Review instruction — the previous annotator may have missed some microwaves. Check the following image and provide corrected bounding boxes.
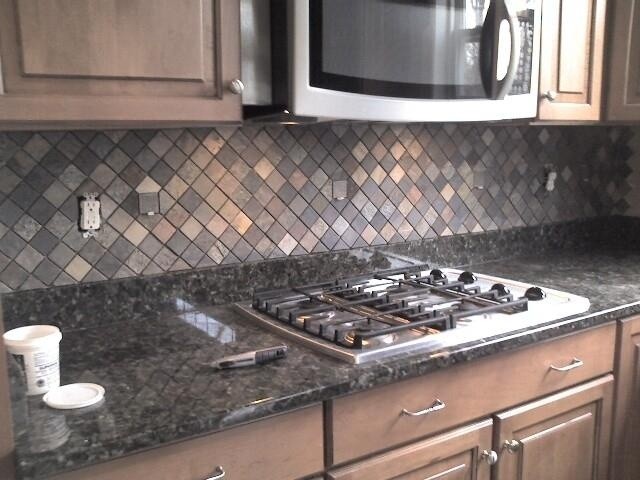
[251,0,543,125]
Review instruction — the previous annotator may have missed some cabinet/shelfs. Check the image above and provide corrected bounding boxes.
[329,322,616,480]
[1,0,246,130]
[70,400,325,476]
[528,0,638,127]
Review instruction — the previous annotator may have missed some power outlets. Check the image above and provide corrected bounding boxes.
[77,195,104,234]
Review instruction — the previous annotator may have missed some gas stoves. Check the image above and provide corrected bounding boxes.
[230,264,591,366]
